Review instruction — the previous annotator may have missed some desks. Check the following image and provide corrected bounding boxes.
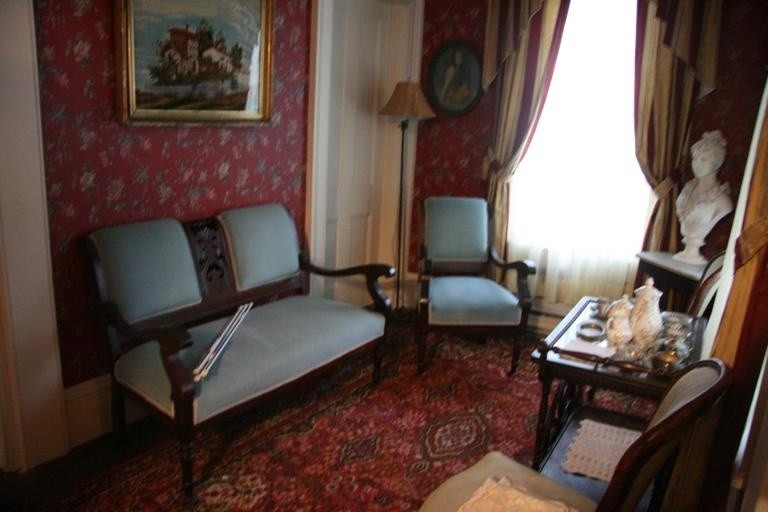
[637,251,707,297]
[528,294,707,501]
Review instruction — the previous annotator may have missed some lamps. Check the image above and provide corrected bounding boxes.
[373,80,436,323]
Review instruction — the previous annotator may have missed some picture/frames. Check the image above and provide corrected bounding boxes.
[425,40,484,117]
[110,1,275,127]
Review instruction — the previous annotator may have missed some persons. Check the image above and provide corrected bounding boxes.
[675,130,733,246]
[439,49,471,110]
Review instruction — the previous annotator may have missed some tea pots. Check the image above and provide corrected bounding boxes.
[630,277,663,346]
[604,292,634,348]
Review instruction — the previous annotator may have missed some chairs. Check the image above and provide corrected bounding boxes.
[413,194,536,378]
[413,357,729,511]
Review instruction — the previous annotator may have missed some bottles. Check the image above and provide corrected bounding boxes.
[654,312,694,374]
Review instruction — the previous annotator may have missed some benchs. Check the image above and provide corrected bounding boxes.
[77,199,397,502]
